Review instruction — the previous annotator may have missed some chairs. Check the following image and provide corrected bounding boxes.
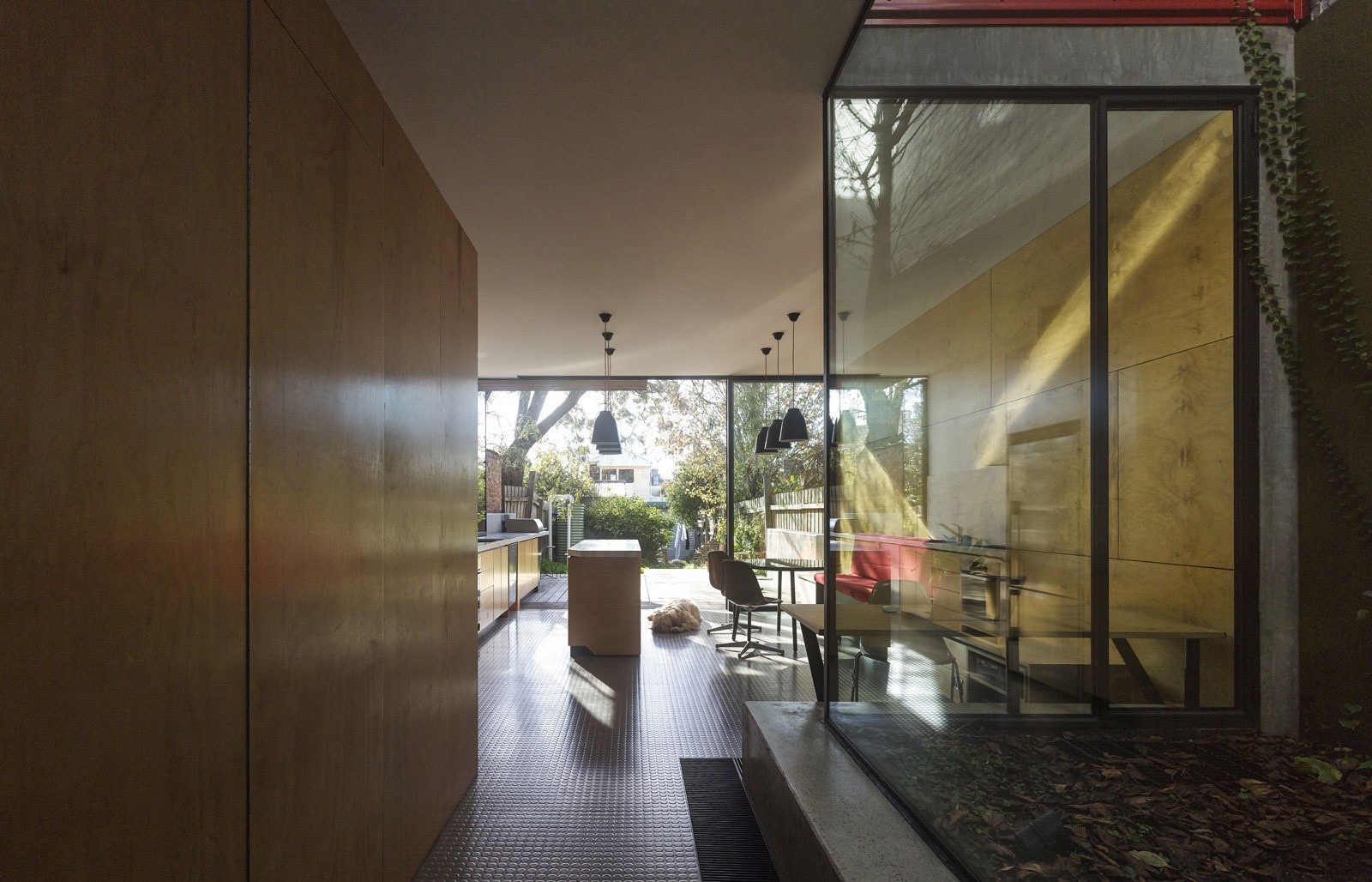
[707,551,762,641]
[850,580,963,705]
[715,559,785,658]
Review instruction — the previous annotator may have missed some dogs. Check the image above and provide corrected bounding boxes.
[648,598,703,633]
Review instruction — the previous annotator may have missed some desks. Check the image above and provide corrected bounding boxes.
[736,557,842,653]
[779,604,1228,712]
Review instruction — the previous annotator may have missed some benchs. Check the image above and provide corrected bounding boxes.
[814,532,943,605]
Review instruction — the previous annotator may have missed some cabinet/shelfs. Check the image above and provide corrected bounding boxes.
[477,544,510,634]
[512,538,538,610]
[899,544,1007,656]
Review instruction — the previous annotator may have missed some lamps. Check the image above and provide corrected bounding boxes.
[590,313,623,456]
[778,312,808,442]
[764,332,791,451]
[754,347,779,455]
